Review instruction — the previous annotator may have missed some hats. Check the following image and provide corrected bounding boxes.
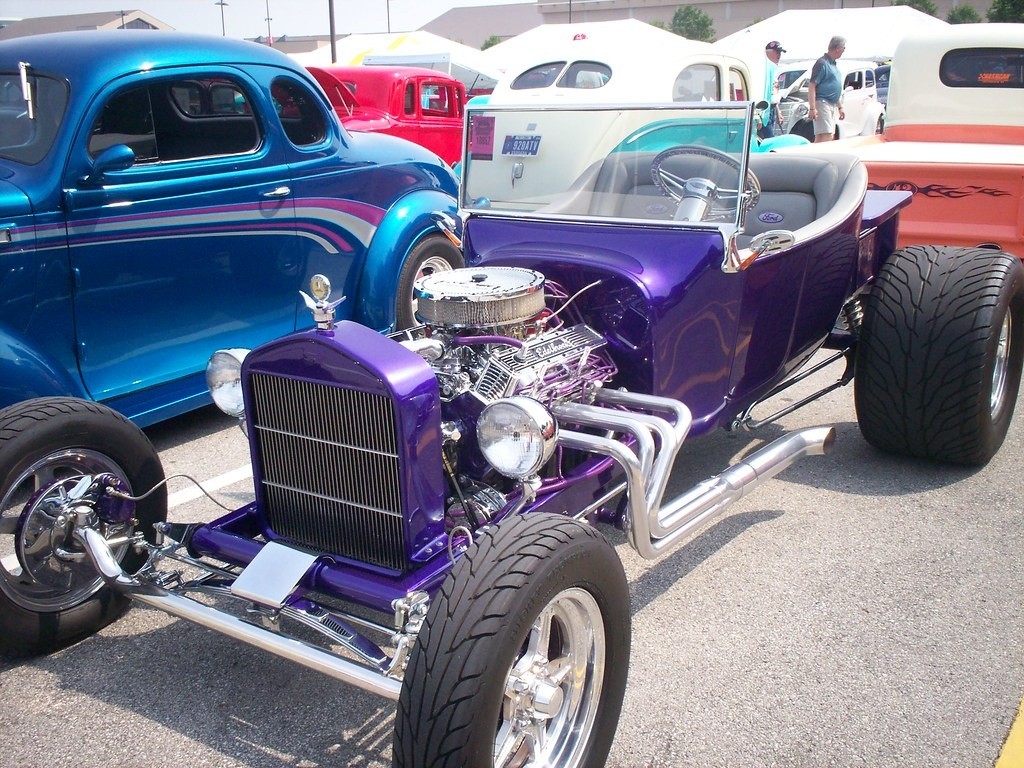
[766,41,787,53]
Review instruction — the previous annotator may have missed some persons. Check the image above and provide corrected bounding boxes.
[751,41,786,140]
[808,36,846,144]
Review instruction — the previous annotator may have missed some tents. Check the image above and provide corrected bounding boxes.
[283,4,953,75]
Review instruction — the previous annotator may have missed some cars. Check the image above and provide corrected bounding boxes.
[1,92,1022,768]
[286,15,1024,291]
[1,27,492,481]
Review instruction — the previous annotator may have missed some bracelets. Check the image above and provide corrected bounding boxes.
[839,107,844,110]
[810,109,815,110]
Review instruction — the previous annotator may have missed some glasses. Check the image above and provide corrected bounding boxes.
[841,47,845,49]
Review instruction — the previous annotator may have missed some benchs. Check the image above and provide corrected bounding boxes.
[592,149,868,256]
